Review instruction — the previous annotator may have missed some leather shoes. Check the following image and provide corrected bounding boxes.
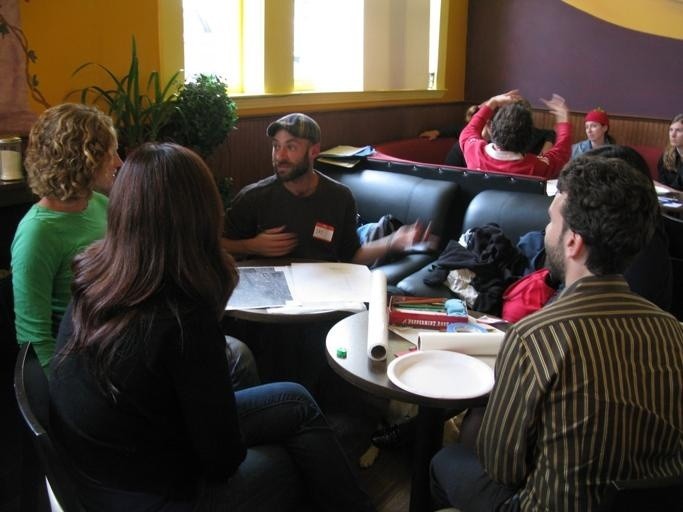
[371,417,418,449]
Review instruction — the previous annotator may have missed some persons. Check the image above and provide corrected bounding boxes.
[568,107,616,162]
[656,112,683,191]
[442,104,490,168]
[457,89,573,181]
[47,140,381,512]
[218,109,434,415]
[10,101,262,393]
[429,156,683,510]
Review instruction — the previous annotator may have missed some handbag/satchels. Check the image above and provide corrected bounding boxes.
[502,268,556,323]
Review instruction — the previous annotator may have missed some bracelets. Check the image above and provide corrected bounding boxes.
[385,231,394,255]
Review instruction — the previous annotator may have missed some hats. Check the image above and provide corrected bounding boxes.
[267,113,321,143]
[584,108,609,125]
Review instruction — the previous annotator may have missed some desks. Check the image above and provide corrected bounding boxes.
[205,248,371,321]
[325,302,519,512]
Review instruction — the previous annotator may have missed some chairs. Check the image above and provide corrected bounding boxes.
[12,339,98,512]
[312,171,554,299]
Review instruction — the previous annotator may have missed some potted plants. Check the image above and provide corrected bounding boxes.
[62,33,184,162]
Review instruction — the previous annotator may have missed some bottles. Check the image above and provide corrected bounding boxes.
[1,134,25,182]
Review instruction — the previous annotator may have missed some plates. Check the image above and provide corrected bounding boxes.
[387,349,496,401]
